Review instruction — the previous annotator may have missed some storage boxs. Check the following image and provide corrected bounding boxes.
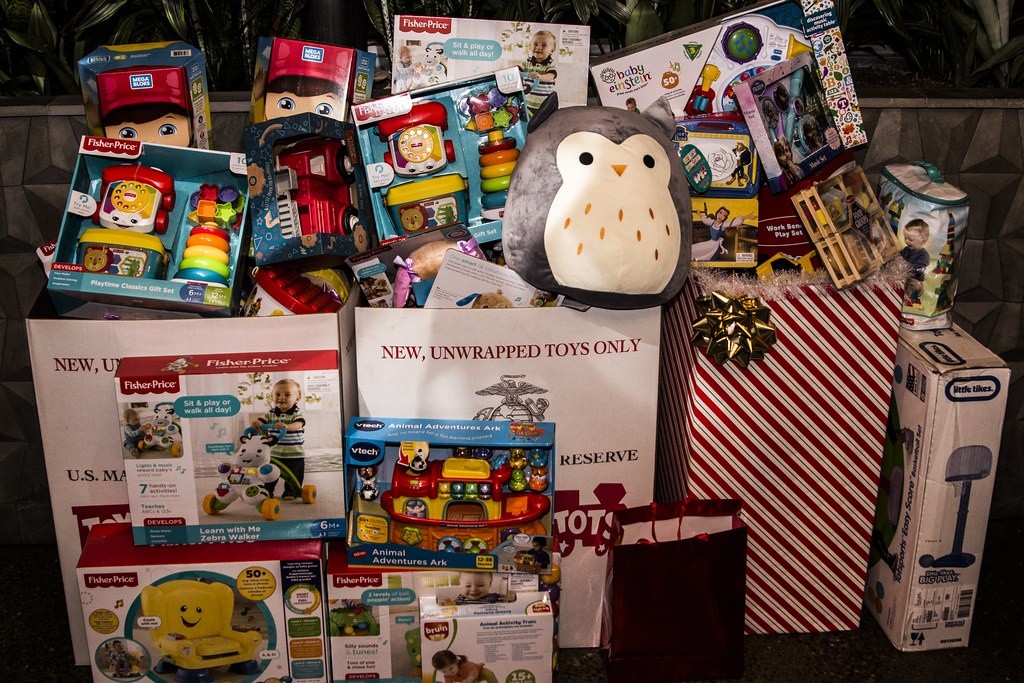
[24,0,1013,683]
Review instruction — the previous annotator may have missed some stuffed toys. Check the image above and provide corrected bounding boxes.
[500,91,693,310]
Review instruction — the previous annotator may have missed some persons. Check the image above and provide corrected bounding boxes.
[111,640,136,676]
[518,536,551,569]
[453,571,508,606]
[394,47,422,94]
[432,650,487,683]
[898,218,931,300]
[516,31,558,120]
[123,409,160,459]
[252,379,306,501]
[626,98,641,115]
